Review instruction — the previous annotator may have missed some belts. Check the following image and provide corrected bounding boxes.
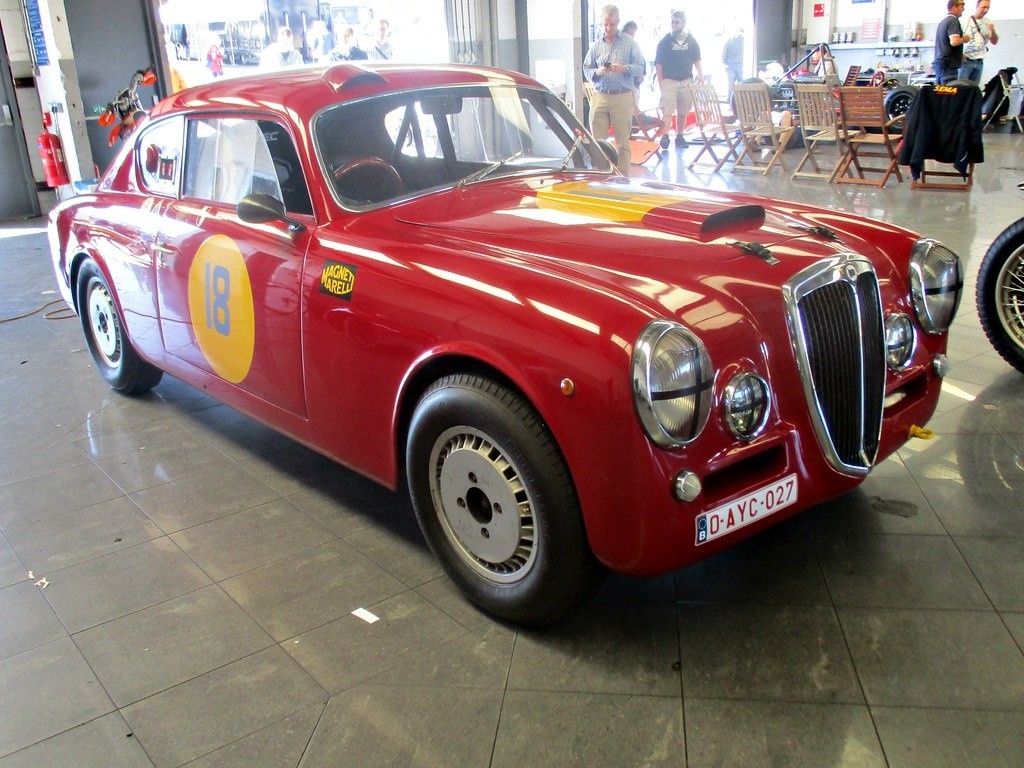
[596,88,630,94]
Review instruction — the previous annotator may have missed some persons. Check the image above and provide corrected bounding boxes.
[721,25,743,103]
[621,20,647,134]
[958,0,999,85]
[931,0,971,85]
[205,45,225,79]
[582,4,645,177]
[653,11,704,149]
[810,43,838,77]
[260,19,397,70]
[746,110,821,155]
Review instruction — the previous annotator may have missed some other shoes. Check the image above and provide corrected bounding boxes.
[771,142,785,154]
[751,141,762,153]
[675,134,689,148]
[661,135,670,149]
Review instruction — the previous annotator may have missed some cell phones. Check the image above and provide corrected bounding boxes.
[604,62,611,68]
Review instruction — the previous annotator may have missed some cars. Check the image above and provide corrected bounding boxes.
[47,62,964,626]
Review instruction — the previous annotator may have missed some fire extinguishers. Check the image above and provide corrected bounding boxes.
[36,124,69,186]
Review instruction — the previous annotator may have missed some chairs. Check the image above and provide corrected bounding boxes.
[687,82,751,173]
[318,110,428,200]
[981,67,1024,135]
[790,83,861,184]
[582,81,665,162]
[842,65,861,86]
[835,86,908,188]
[730,83,795,176]
[909,85,979,192]
[226,133,311,215]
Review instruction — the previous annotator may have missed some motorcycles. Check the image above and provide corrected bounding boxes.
[98,64,159,147]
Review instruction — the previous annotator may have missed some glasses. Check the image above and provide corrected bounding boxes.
[672,20,679,25]
[956,2,965,6]
